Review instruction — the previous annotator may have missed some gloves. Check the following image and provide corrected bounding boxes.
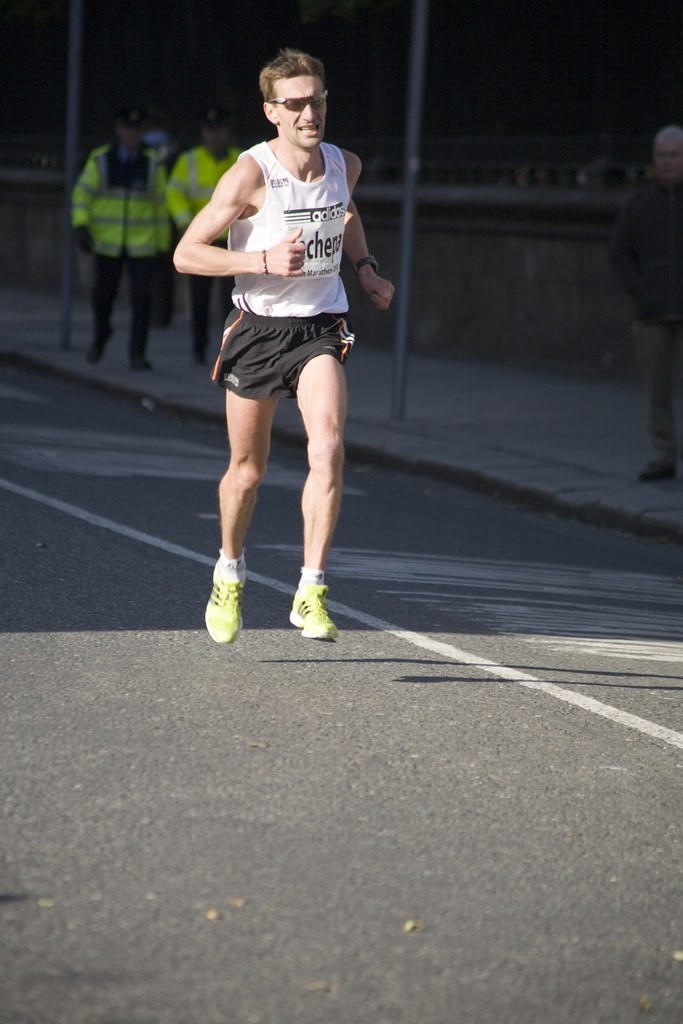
[76,226,95,254]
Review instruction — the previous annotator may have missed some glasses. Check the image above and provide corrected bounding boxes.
[267,89,328,111]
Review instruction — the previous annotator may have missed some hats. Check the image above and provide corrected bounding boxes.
[193,104,232,127]
[112,105,147,127]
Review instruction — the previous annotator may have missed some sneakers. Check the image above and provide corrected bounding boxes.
[205,559,247,644]
[289,583,338,639]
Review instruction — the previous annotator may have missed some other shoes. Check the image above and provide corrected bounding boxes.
[129,356,150,370]
[192,333,209,364]
[640,464,677,480]
[86,328,113,363]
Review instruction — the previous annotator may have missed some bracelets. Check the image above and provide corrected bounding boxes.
[354,255,378,272]
[262,250,268,275]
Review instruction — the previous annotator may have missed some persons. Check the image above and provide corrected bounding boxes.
[173,52,395,643]
[613,127,683,482]
[69,110,169,370]
[164,112,245,367]
[141,130,180,330]
